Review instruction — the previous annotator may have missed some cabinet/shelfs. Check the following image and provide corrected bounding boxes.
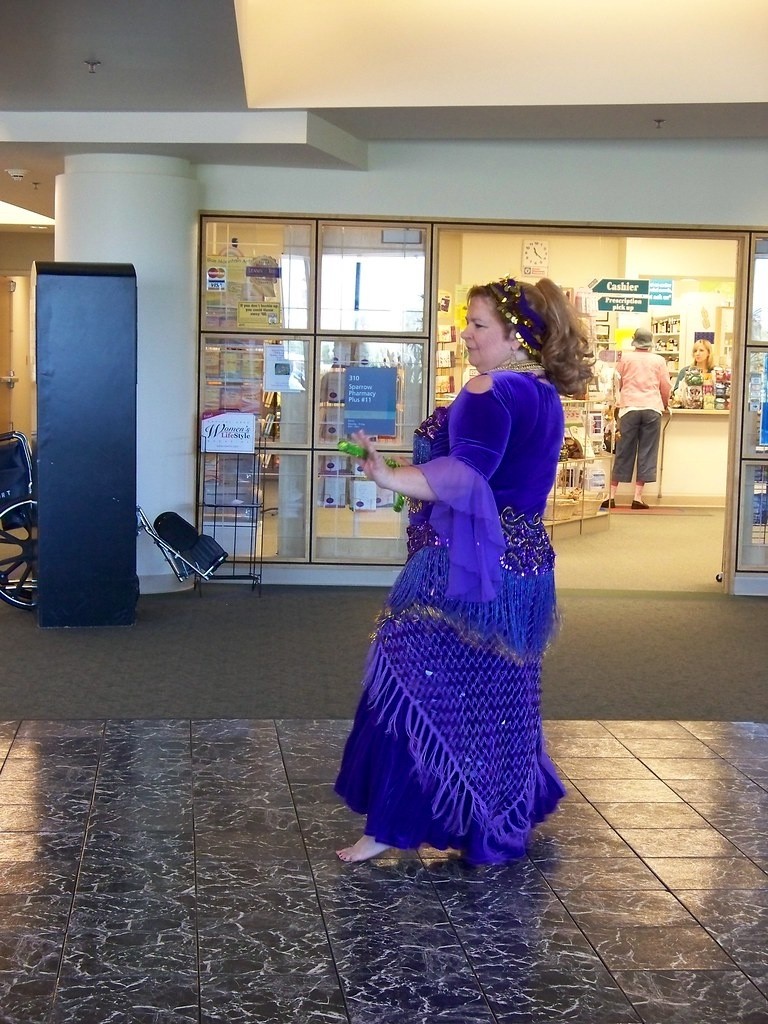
[542,342,617,541]
[193,434,267,600]
[203,296,263,556]
[650,311,696,375]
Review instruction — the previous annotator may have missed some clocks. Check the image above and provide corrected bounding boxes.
[521,240,549,278]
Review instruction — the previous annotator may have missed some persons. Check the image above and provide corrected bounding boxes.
[333,276,599,865]
[672,339,720,394]
[601,328,671,510]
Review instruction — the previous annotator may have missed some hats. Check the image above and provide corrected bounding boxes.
[629,327,654,348]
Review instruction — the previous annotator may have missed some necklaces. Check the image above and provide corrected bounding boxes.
[493,360,545,371]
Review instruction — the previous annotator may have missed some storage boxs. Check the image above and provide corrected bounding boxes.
[436,324,456,344]
[205,280,263,523]
[321,372,345,403]
[318,455,347,476]
[200,409,260,456]
[436,350,456,368]
[435,376,455,394]
[347,457,366,481]
[349,480,377,513]
[319,406,347,444]
[377,486,394,508]
[317,477,346,509]
[348,433,380,444]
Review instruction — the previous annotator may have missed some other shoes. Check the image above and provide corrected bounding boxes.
[632,499,650,509]
[601,499,616,509]
